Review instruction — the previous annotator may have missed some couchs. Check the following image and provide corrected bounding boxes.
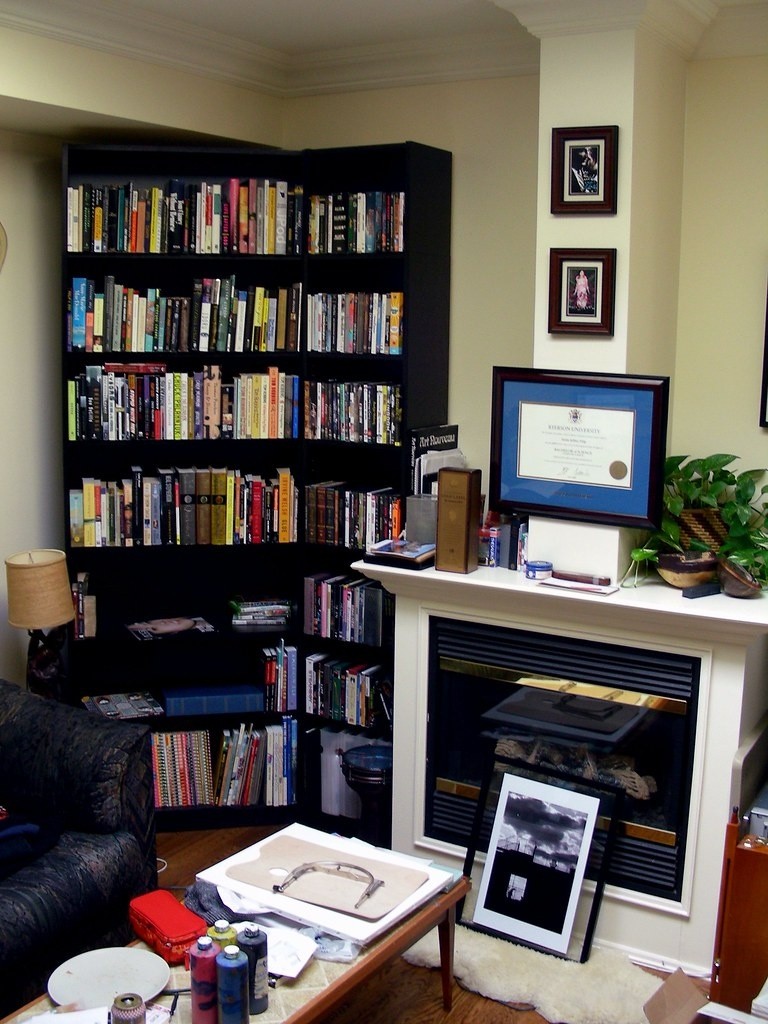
[0,682,156,1023]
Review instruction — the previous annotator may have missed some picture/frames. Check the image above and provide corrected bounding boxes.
[550,126,618,217]
[453,752,625,966]
[548,246,617,337]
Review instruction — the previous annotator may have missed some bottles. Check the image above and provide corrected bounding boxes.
[189,920,269,1024]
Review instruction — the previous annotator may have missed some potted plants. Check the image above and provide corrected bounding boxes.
[626,453,768,589]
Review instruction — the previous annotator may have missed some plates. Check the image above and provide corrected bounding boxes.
[47,946,171,1009]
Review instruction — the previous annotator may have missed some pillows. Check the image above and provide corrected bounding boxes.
[0,798,55,881]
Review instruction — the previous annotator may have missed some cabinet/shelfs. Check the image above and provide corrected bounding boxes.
[64,130,457,833]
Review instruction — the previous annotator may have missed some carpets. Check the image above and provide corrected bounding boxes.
[404,924,663,1024]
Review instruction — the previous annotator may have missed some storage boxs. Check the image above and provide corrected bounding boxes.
[434,467,482,575]
[406,491,437,543]
[129,891,208,970]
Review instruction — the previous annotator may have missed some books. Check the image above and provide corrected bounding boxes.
[230,598,291,625]
[68,363,403,446]
[126,616,219,641]
[67,177,405,255]
[304,571,396,646]
[151,714,297,807]
[70,466,400,556]
[81,691,164,720]
[71,572,96,639]
[362,424,464,570]
[263,638,393,742]
[489,513,528,573]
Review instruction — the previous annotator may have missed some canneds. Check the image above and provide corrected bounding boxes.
[110,993,147,1024]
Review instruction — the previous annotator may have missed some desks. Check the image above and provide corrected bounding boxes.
[0,866,472,1024]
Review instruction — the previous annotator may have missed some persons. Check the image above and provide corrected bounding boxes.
[573,270,590,309]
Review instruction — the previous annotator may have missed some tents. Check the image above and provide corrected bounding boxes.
[67,275,403,354]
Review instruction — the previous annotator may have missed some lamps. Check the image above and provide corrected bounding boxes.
[4,549,73,698]
